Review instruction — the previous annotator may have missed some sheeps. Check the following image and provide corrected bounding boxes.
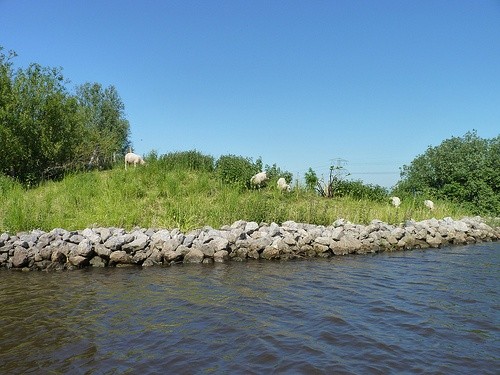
[390,196,403,208]
[424,198,435,211]
[124,152,146,171]
[251,171,270,190]
[276,177,293,195]
